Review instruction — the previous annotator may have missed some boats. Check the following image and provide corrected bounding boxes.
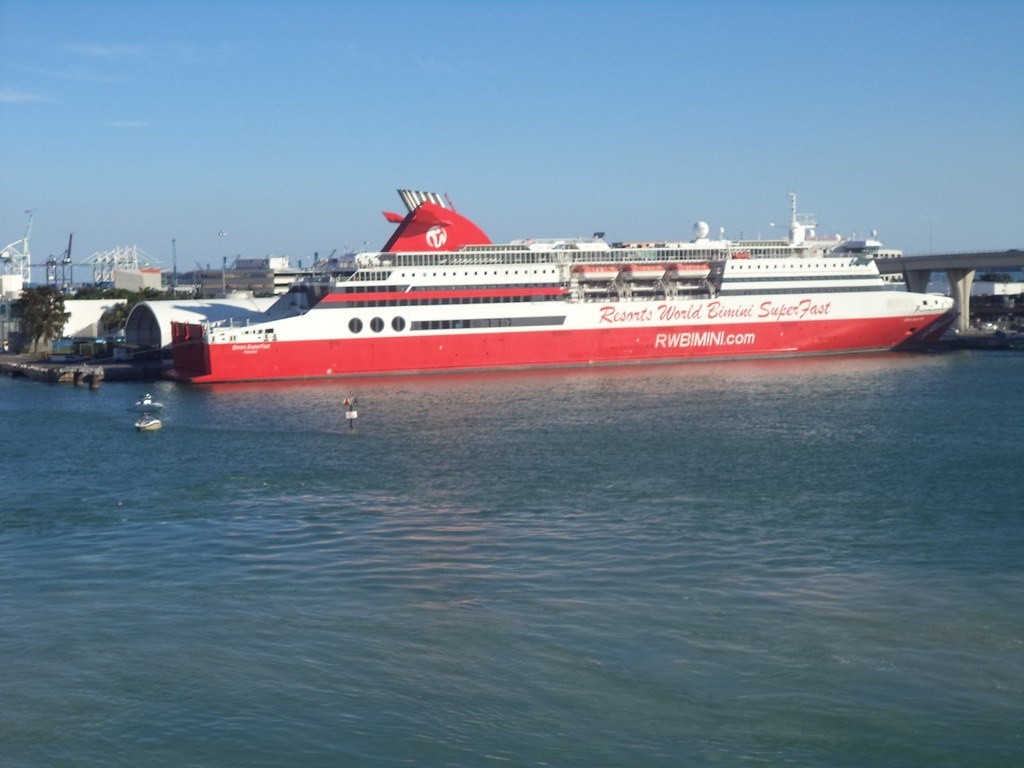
[151,189,955,387]
[135,412,163,431]
[129,392,163,413]
